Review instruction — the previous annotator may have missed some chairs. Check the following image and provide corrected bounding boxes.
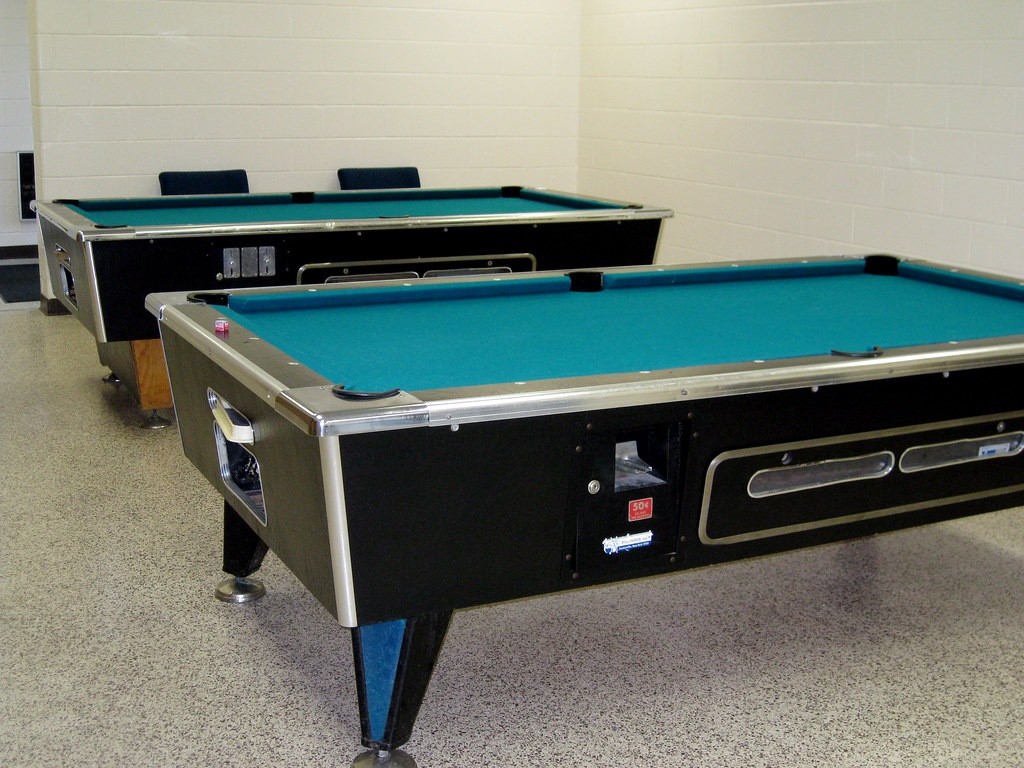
[338,164,423,191]
[158,167,248,197]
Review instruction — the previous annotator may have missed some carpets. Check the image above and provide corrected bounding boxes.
[0,263,40,304]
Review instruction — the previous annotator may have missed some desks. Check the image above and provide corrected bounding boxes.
[23,182,676,430]
[140,247,1024,768]
